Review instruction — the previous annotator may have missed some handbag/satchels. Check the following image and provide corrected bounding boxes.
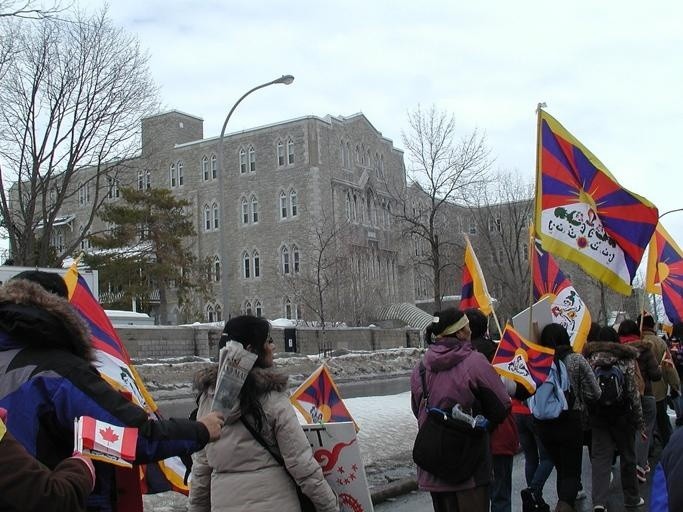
[412,409,486,483]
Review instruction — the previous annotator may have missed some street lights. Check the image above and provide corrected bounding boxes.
[217,75,295,325]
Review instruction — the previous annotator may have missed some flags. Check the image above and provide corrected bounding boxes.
[60,264,191,494]
[290,363,359,433]
[73,412,141,470]
[529,223,593,355]
[535,107,659,297]
[458,244,495,319]
[644,219,683,326]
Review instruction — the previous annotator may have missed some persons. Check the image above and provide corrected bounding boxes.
[0,407,98,512]
[183,315,340,511]
[1,268,227,512]
[408,304,683,511]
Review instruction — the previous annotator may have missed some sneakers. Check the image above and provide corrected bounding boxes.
[521,488,608,512]
[624,497,645,506]
[636,463,651,482]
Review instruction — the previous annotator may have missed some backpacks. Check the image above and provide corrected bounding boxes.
[527,358,575,421]
[589,359,628,415]
